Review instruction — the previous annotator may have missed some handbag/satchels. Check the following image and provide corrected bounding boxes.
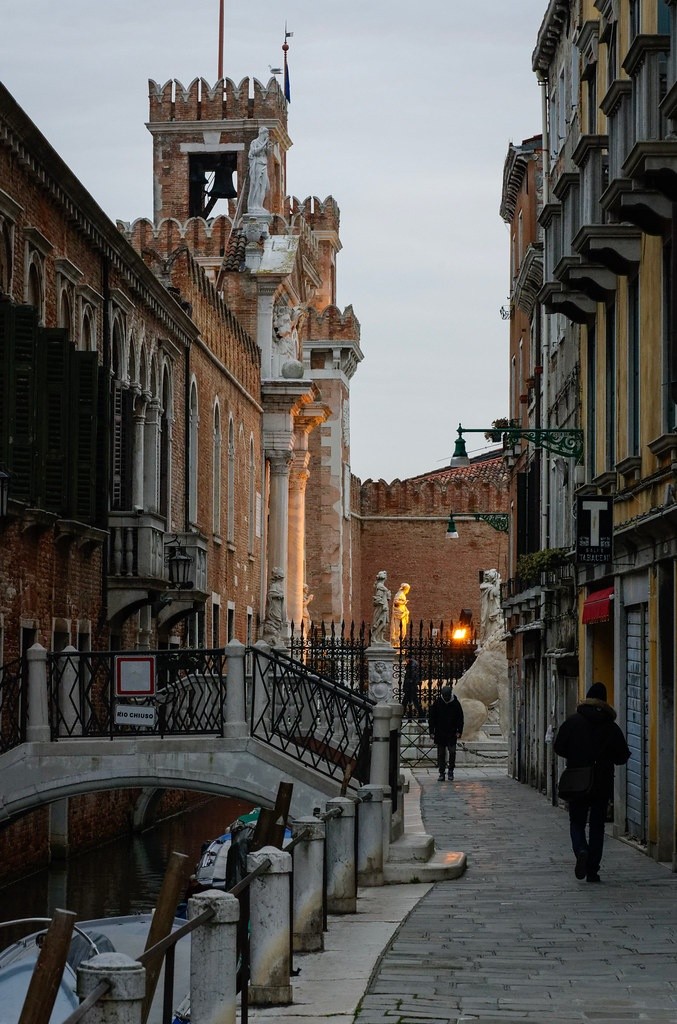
[558,766,600,802]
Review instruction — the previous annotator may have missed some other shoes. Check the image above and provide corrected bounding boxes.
[448,771,454,780]
[587,875,600,882]
[437,772,446,780]
[575,850,588,879]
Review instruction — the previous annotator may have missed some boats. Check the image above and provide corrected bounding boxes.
[0,912,194,1023]
[193,805,295,893]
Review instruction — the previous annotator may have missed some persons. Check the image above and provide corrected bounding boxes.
[477,568,504,646]
[273,305,300,377]
[372,571,410,647]
[401,654,425,721]
[247,126,272,215]
[428,686,465,781]
[553,682,632,881]
[301,583,313,640]
[262,567,286,650]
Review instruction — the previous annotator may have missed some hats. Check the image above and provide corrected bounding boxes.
[586,682,607,703]
